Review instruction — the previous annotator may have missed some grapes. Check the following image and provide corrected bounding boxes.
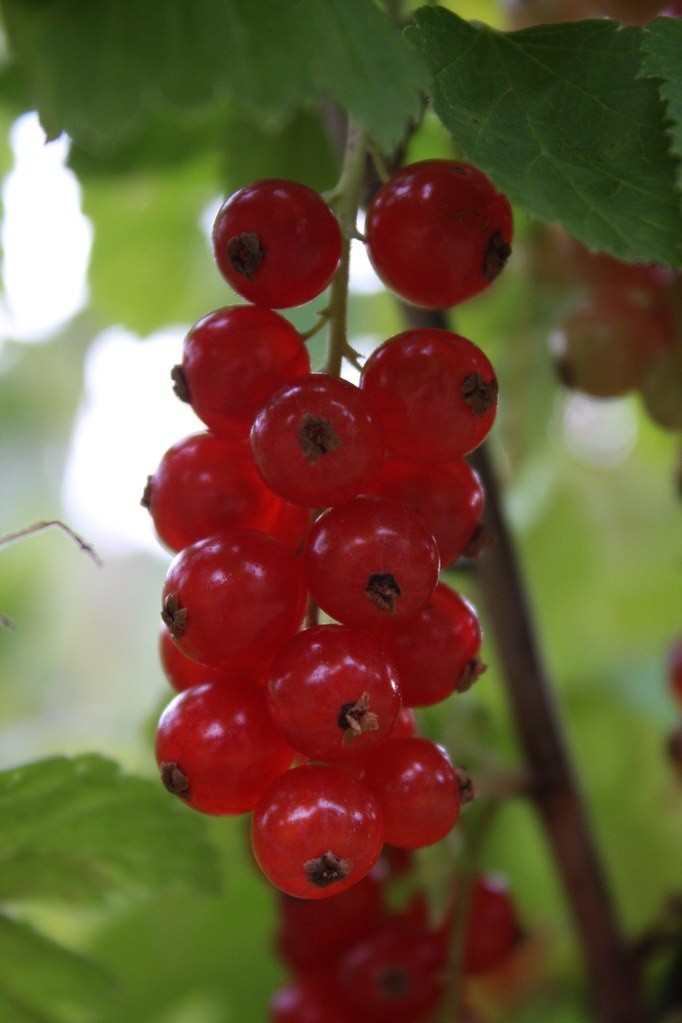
[141,156,682,1022]
